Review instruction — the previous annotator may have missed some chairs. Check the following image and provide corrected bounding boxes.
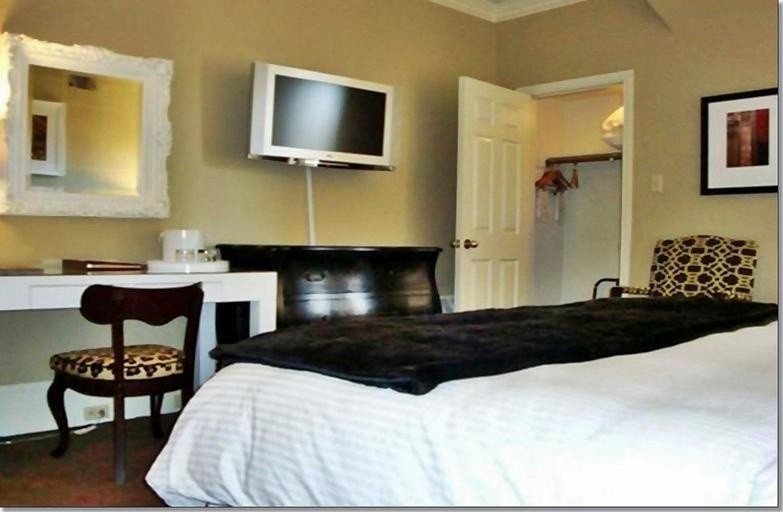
[43,284,203,487]
[608,233,758,302]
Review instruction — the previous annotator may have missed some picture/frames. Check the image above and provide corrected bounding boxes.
[701,86,777,194]
[31,100,65,176]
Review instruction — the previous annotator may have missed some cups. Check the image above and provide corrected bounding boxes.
[197,249,222,265]
[175,249,195,265]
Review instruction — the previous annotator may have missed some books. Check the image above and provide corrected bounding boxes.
[62,259,147,272]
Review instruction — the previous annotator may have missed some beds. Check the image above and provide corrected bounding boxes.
[143,297,778,506]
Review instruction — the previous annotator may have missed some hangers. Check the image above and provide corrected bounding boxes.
[535,166,580,198]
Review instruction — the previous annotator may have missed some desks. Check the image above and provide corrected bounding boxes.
[0,268,279,392]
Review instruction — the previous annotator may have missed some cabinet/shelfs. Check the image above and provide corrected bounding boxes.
[209,243,442,372]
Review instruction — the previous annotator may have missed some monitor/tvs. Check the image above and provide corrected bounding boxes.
[249,61,401,168]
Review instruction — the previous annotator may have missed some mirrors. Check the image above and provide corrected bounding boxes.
[1,29,175,219]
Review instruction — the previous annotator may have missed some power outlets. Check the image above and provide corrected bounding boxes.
[83,403,109,422]
